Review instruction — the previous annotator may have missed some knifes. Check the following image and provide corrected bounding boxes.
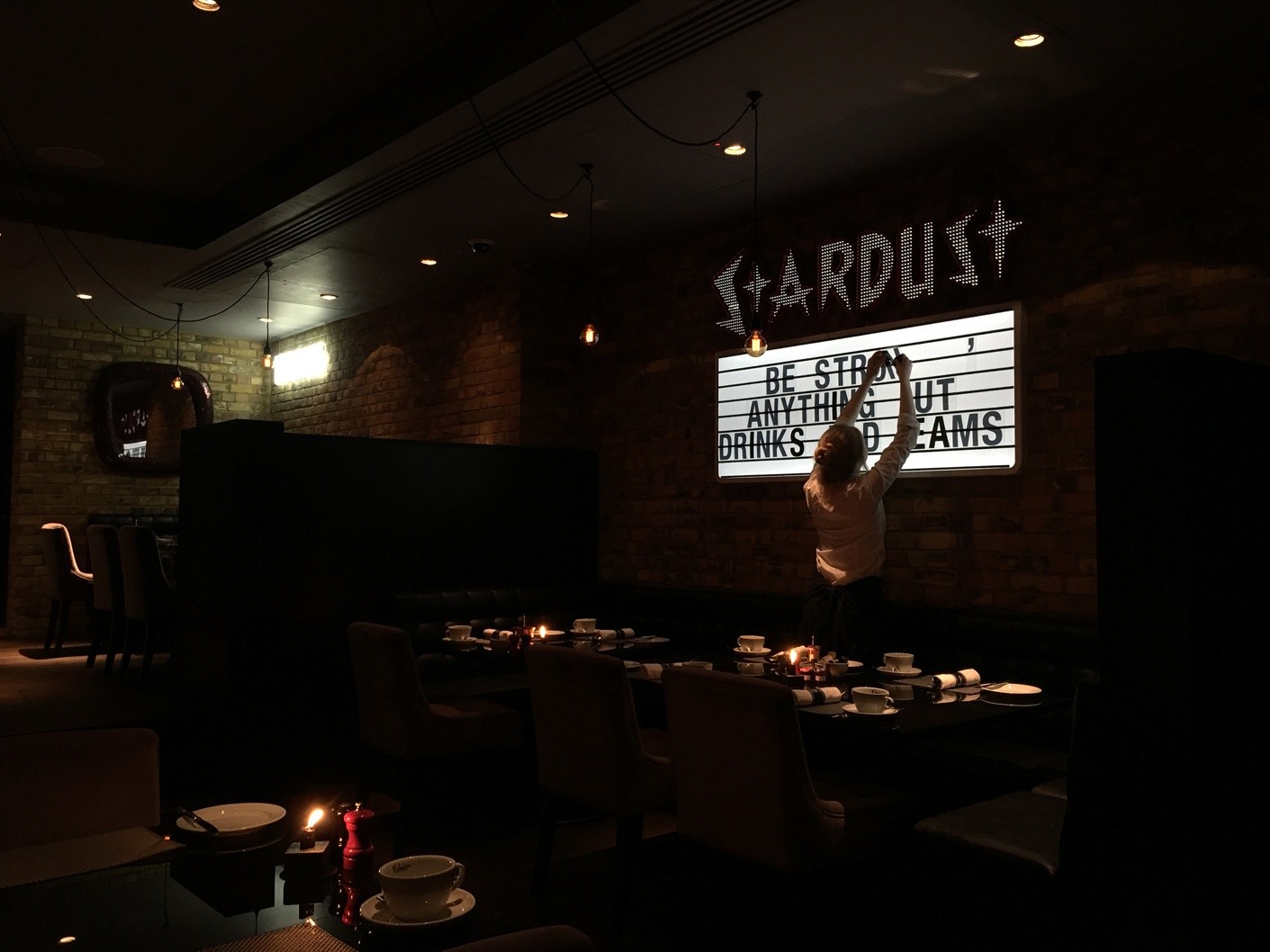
[175,806,219,833]
[975,680,1009,688]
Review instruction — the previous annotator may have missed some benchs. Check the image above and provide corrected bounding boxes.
[88,511,178,647]
[365,583,1100,952]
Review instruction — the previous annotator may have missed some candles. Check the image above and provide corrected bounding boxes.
[300,803,326,849]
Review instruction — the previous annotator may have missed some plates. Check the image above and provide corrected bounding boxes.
[842,703,899,715]
[733,656,778,664]
[980,683,1042,695]
[732,647,772,656]
[632,636,671,643]
[876,666,922,677]
[570,629,600,636]
[978,693,1043,707]
[817,660,864,668]
[878,675,915,687]
[442,637,477,641]
[176,802,286,837]
[359,888,476,926]
[621,660,641,668]
[533,630,565,638]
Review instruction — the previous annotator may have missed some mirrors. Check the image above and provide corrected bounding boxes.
[96,361,214,476]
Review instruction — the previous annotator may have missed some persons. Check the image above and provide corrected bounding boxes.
[802,351,918,657]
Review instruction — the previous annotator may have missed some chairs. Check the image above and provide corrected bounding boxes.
[0,727,161,851]
[41,523,178,689]
[348,620,925,952]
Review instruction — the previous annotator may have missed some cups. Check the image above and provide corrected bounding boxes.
[737,662,764,676]
[737,635,765,652]
[378,854,466,922]
[884,684,914,700]
[883,652,914,672]
[445,625,472,640]
[573,619,596,632]
[851,686,895,712]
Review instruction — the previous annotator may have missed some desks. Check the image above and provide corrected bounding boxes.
[412,631,1080,795]
[0,797,493,952]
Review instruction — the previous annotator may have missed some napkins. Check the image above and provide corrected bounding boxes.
[640,660,682,679]
[792,685,839,706]
[597,627,634,641]
[934,668,982,693]
[481,626,513,639]
